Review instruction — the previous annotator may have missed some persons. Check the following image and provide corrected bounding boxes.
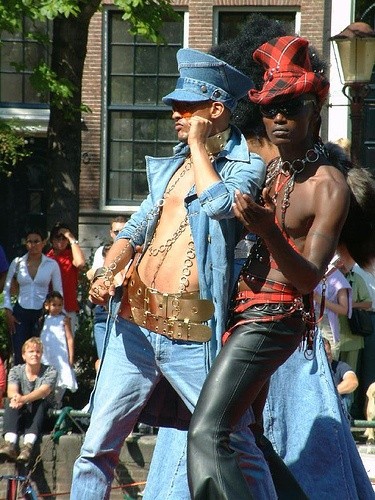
[68,47,278,500]
[333,256,372,372]
[311,261,348,360]
[336,241,375,420]
[0,337,58,463]
[40,291,78,409]
[87,215,133,375]
[45,227,85,339]
[322,337,358,425]
[2,229,63,365]
[187,36,353,500]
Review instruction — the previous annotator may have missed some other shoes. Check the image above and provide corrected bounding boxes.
[17,443,33,464]
[0,440,18,460]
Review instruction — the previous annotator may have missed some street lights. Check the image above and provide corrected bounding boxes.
[327,22,375,167]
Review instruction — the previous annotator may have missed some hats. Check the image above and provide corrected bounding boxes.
[162,48,256,114]
[248,35,330,104]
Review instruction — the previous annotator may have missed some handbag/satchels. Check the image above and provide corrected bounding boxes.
[10,257,20,297]
[347,308,375,337]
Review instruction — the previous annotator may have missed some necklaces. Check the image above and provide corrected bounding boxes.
[93,124,235,337]
[234,136,335,292]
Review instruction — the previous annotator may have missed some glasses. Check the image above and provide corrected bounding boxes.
[111,230,120,236]
[26,239,42,246]
[52,234,67,240]
[170,98,212,115]
[259,97,316,118]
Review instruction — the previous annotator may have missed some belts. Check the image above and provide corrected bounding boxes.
[119,266,215,343]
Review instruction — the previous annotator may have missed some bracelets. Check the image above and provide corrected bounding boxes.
[69,239,79,244]
[92,267,114,285]
